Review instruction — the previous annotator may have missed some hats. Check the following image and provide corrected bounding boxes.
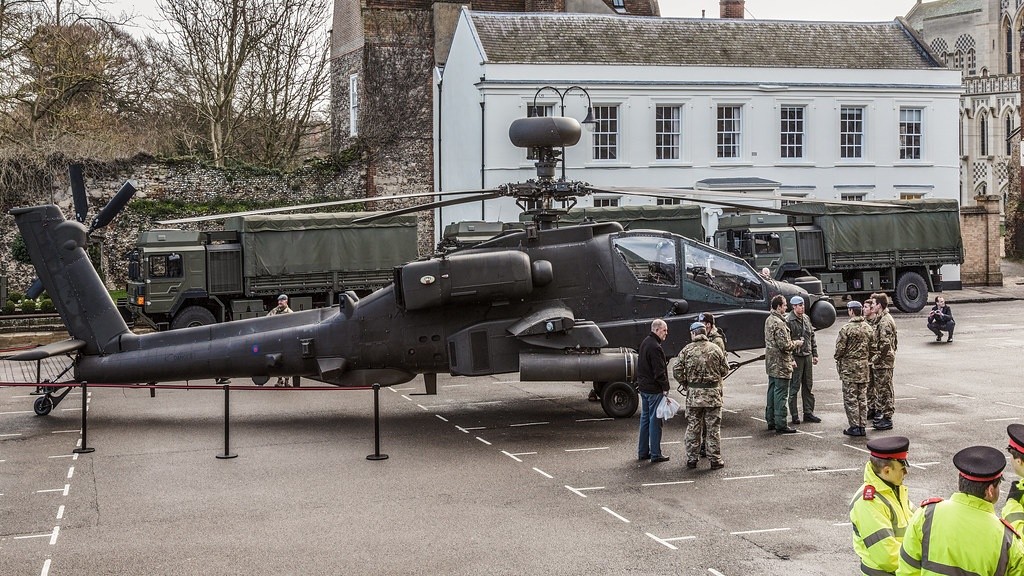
[690,322,706,331]
[867,436,910,468]
[953,446,1007,482]
[790,296,804,304]
[847,301,863,308]
[277,295,288,300]
[1005,423,1024,454]
[699,313,715,324]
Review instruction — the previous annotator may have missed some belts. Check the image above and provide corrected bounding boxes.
[688,381,719,388]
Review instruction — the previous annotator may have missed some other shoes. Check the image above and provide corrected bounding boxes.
[947,337,953,342]
[792,416,800,424]
[803,413,821,423]
[711,461,724,470]
[936,333,944,341]
[873,417,893,429]
[687,460,696,468]
[768,425,776,430]
[876,410,882,416]
[867,409,877,419]
[639,454,651,459]
[275,383,292,387]
[651,456,670,462]
[843,427,866,436]
[776,427,796,433]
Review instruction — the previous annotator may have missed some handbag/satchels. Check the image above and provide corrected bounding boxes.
[656,396,680,418]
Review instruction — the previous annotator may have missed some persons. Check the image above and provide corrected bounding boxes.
[895,446,1024,576]
[849,436,918,576]
[266,294,294,387]
[1001,424,1024,545]
[765,295,821,433]
[673,312,730,470]
[638,319,670,462]
[927,296,955,342]
[834,293,897,436]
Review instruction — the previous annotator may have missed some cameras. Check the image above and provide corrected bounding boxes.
[931,310,940,314]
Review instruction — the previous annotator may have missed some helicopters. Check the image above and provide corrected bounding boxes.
[8,116,831,416]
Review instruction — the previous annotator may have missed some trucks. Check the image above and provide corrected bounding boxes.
[713,197,965,314]
[126,210,420,331]
[436,204,706,253]
